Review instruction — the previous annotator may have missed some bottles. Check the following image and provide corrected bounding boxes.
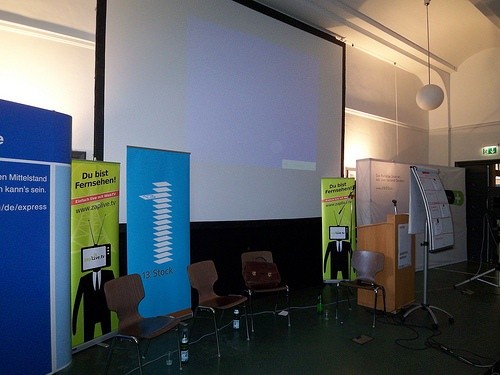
[316,293,323,315]
[181,332,190,363]
[232,306,240,330]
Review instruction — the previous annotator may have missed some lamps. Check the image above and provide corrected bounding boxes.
[415,0,445,112]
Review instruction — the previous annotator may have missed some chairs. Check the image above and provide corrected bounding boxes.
[333,249,389,330]
[240,251,291,332]
[186,260,251,357]
[105,273,181,375]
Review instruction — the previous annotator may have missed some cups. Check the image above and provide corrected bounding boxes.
[323,308,329,320]
[166,350,173,365]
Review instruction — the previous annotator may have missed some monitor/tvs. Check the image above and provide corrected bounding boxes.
[329,210,348,240]
[80,215,111,272]
